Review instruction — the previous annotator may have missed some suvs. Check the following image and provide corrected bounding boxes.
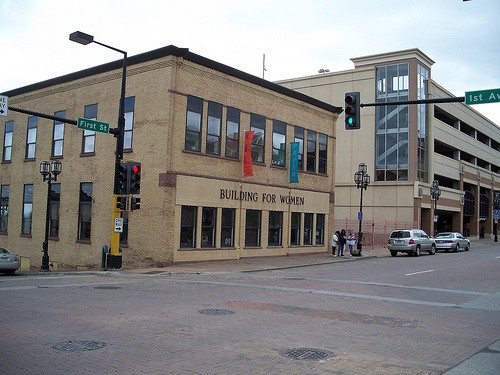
[386,228,436,257]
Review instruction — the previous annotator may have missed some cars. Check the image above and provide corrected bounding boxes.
[434,232,471,253]
[0,247,20,274]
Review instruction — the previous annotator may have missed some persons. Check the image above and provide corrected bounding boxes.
[348,232,356,256]
[338,229,347,256]
[331,231,339,256]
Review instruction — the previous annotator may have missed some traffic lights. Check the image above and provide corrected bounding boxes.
[126,161,142,195]
[344,92,360,130]
[116,196,127,212]
[113,162,127,195]
[128,197,141,212]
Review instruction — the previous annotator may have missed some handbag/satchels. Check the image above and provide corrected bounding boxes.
[347,239,357,246]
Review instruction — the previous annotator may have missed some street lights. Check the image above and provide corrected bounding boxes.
[493,194,500,242]
[39,160,63,272]
[354,162,371,256]
[430,180,442,238]
[69,30,128,270]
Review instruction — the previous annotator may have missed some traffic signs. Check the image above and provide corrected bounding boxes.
[114,218,123,233]
[0,95,8,117]
